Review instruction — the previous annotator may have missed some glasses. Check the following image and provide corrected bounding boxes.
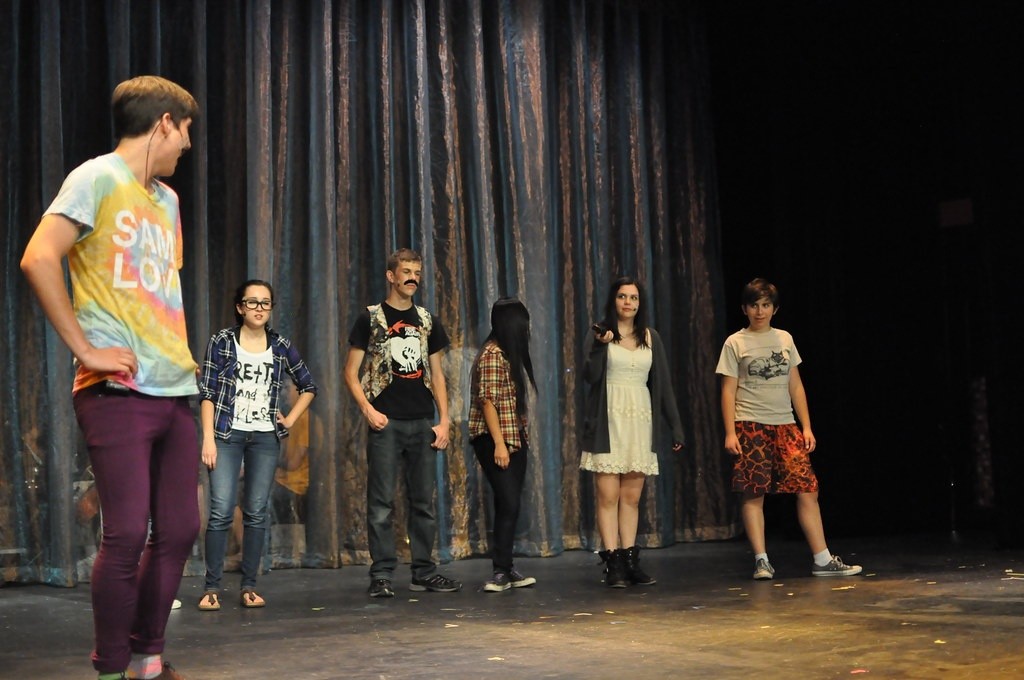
[241,298,273,311]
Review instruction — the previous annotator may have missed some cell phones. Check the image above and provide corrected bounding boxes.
[591,324,606,336]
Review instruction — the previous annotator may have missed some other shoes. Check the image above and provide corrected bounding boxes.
[128,661,187,680]
[171,599,181,609]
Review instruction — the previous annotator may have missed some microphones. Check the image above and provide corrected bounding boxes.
[633,308,637,311]
[397,280,400,285]
[243,312,246,316]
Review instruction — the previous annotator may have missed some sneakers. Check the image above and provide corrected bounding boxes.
[369,579,396,597]
[812,554,867,577]
[482,572,512,591]
[754,559,774,579]
[509,570,537,587]
[408,570,462,591]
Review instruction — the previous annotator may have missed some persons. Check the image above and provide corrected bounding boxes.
[714,279,865,580]
[345,249,461,597]
[576,278,687,587]
[199,280,317,609]
[20,74,200,680]
[469,296,537,592]
[232,379,323,558]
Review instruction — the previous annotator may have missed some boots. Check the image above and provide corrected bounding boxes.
[628,545,657,586]
[599,548,630,589]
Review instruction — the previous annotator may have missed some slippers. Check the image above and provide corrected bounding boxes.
[240,585,266,608]
[199,590,221,611]
